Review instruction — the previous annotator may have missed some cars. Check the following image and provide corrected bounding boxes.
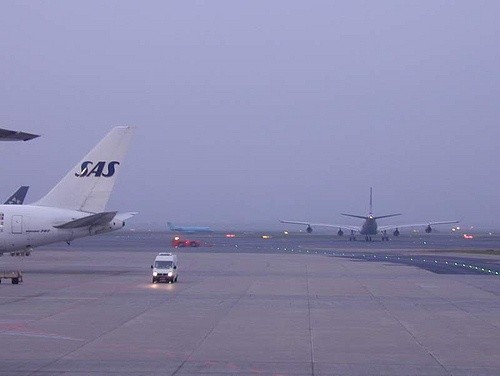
[174,235,200,248]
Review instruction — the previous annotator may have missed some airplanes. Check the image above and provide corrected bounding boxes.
[1,124,139,284]
[166,222,215,235]
[279,187,459,244]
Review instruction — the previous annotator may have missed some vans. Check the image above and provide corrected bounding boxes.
[150,252,178,283]
